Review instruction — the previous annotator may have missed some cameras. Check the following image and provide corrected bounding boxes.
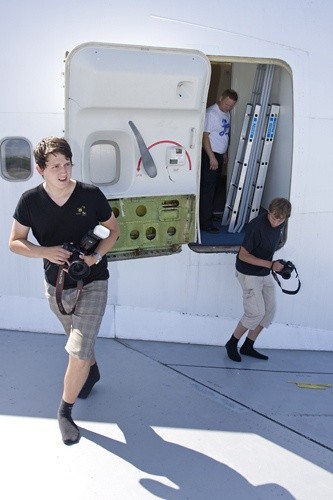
[62,224,110,282]
[276,259,295,279]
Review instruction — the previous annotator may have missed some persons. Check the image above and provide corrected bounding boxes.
[8,135,121,445]
[199,89,238,234]
[225,198,294,362]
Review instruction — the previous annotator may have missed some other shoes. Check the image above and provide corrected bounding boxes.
[208,214,222,222]
[200,225,220,233]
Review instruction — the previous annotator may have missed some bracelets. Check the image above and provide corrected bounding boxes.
[270,261,276,270]
[93,251,102,264]
